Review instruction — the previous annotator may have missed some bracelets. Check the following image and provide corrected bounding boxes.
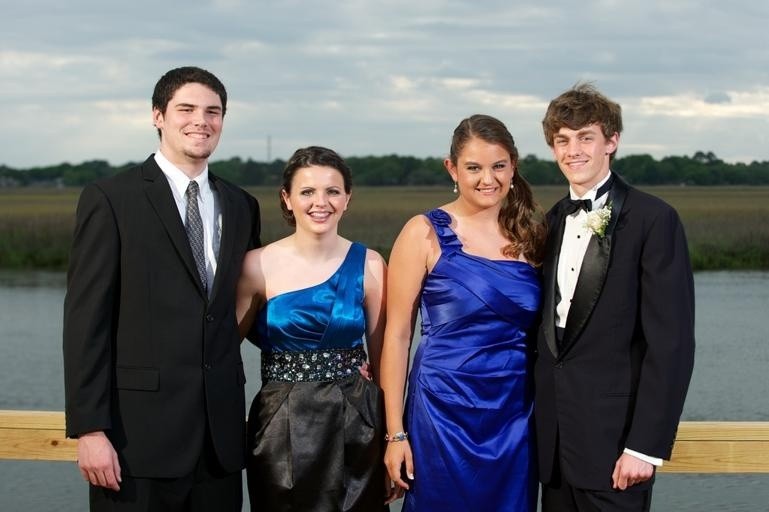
[385,432,408,442]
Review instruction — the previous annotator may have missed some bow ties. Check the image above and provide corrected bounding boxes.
[562,198,591,215]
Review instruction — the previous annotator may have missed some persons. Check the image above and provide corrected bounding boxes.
[383,114,549,512]
[236,146,405,512]
[533,81,696,512]
[62,66,374,512]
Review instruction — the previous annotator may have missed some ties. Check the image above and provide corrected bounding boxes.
[184,180,207,294]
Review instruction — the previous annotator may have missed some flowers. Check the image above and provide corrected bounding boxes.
[582,200,615,241]
